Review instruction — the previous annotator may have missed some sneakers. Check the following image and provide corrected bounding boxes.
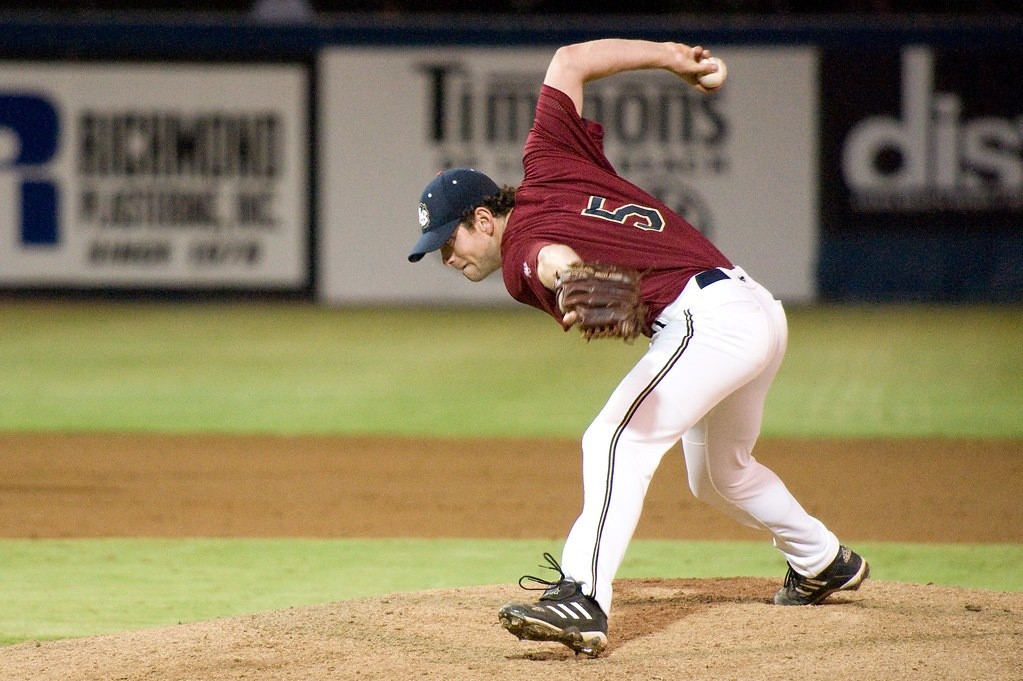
[498,552,609,656]
[774,544,871,606]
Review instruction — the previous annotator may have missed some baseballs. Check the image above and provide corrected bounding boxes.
[697,56,728,90]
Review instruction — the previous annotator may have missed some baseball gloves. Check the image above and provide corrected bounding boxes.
[558,261,645,341]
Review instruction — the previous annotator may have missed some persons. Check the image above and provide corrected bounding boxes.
[409,37,870,657]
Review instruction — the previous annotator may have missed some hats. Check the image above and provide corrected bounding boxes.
[407,168,501,263]
